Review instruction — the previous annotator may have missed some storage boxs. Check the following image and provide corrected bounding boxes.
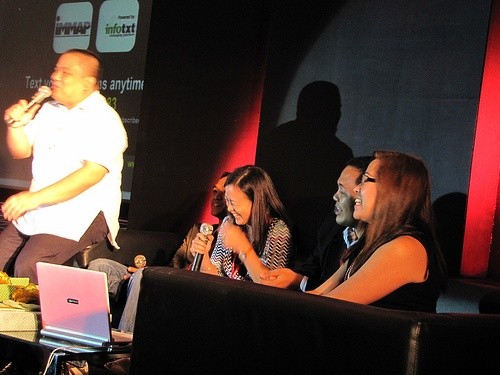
[0,277,38,331]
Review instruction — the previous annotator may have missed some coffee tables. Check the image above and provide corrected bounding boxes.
[0,330,132,375]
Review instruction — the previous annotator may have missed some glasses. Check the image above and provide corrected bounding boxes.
[362,174,377,184]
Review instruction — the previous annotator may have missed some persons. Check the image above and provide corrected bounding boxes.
[305,151,440,313]
[0,47,130,283]
[257,156,374,291]
[190,164,292,283]
[87,172,233,332]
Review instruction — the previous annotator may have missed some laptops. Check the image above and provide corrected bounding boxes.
[36,262,133,349]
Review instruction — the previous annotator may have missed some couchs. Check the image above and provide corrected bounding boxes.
[78,230,500,375]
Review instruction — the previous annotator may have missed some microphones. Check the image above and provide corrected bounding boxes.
[4,86,52,127]
[191,223,213,272]
[135,255,147,269]
